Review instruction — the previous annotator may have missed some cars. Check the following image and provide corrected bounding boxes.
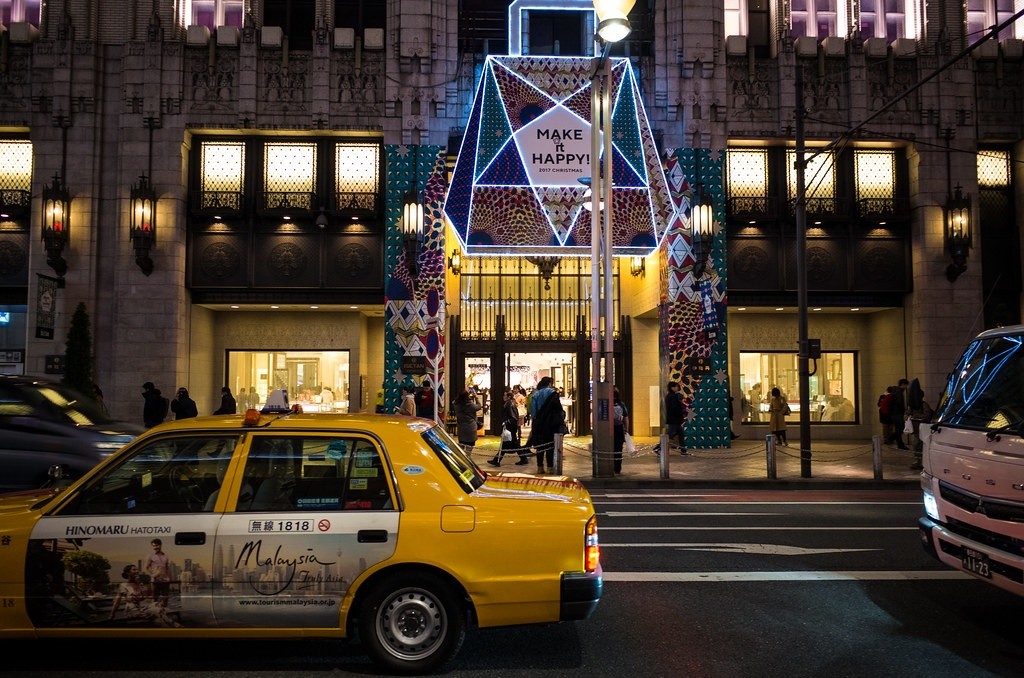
[0,371,173,503]
[0,388,604,678]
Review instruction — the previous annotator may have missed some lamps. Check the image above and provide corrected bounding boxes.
[943,181,972,283]
[447,248,461,276]
[317,213,328,229]
[40,172,71,286]
[130,171,157,275]
[402,144,424,278]
[689,149,713,278]
[630,256,645,276]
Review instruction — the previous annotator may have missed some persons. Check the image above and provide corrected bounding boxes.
[414,381,434,420]
[487,392,528,466]
[613,387,629,474]
[207,387,236,458]
[769,388,789,445]
[653,381,691,456]
[731,396,740,440]
[93,384,103,412]
[395,387,415,416]
[236,387,260,412]
[453,388,481,455]
[512,384,540,456]
[142,382,163,428]
[767,384,788,403]
[749,384,763,421]
[823,394,854,421]
[877,379,911,450]
[171,387,197,420]
[557,385,575,399]
[531,377,567,473]
[320,388,336,403]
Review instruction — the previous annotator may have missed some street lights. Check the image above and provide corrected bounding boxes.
[587,0,640,478]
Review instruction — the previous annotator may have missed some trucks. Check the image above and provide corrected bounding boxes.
[908,324,1024,599]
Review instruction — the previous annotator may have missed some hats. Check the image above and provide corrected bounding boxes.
[422,381,430,387]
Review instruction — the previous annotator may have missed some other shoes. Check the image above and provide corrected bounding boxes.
[537,465,545,474]
[546,466,554,474]
[206,451,219,458]
[895,444,911,451]
[487,459,500,467]
[515,459,528,465]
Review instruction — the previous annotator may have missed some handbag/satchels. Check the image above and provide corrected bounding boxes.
[902,415,915,435]
[781,396,791,415]
[499,422,512,442]
[624,432,635,455]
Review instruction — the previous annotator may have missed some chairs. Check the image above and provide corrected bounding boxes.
[253,443,294,512]
[205,451,253,510]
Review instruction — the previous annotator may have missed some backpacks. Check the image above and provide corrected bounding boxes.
[613,402,624,425]
[880,392,893,415]
